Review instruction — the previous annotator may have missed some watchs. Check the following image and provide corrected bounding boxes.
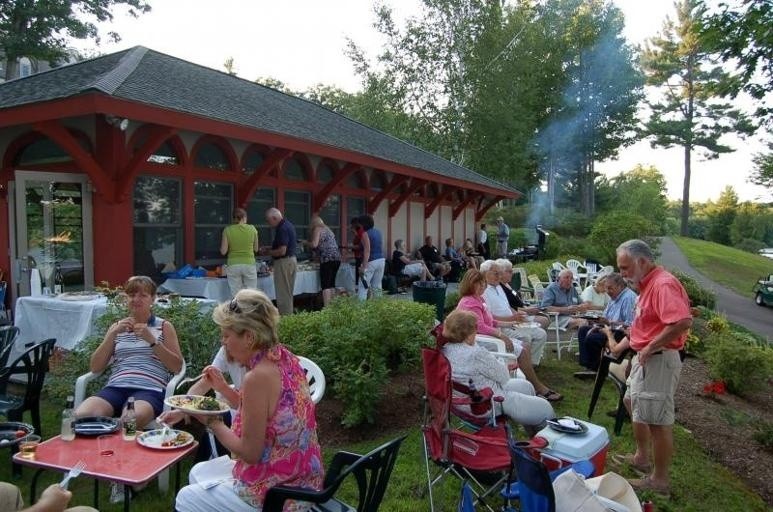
[148,339,161,348]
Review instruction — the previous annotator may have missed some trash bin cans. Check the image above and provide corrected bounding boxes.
[412,281,446,323]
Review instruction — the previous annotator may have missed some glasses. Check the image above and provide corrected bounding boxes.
[229,297,242,314]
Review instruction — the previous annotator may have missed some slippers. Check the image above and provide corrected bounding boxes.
[536,390,564,401]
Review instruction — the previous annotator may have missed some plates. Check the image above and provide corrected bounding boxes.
[138,428,194,450]
[516,322,541,329]
[580,314,601,320]
[549,416,587,433]
[72,416,119,435]
[184,276,195,279]
[60,290,98,302]
[0,421,35,446]
[164,395,230,415]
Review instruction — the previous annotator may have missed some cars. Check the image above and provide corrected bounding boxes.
[758,248,773,260]
[27,239,82,287]
[752,274,773,306]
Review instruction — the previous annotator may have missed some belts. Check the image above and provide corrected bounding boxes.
[632,350,662,355]
[274,254,295,260]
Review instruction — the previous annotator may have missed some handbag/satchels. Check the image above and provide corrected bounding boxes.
[552,467,642,512]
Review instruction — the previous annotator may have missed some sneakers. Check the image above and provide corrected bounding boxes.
[607,407,630,418]
[573,366,597,376]
[108,480,132,503]
[611,452,651,476]
[626,474,669,493]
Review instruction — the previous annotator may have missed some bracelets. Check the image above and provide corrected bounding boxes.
[205,416,222,432]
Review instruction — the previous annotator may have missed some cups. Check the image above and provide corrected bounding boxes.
[43,287,51,297]
[30,268,42,297]
[96,434,116,458]
[215,264,226,277]
[15,434,41,458]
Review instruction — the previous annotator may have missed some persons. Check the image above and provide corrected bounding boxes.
[393,216,510,282]
[444,239,692,488]
[71,273,182,505]
[221,207,296,315]
[302,215,341,291]
[27,238,82,284]
[174,285,327,512]
[0,477,104,512]
[153,341,258,435]
[350,215,386,301]
[132,211,162,275]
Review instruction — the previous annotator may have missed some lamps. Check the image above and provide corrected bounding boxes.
[104,114,129,131]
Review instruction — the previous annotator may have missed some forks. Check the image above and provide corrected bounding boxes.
[176,371,207,389]
[60,459,86,487]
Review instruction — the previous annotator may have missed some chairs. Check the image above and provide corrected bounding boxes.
[262,433,409,512]
[62,354,187,494]
[214,355,327,406]
[0,320,56,455]
[385,251,686,512]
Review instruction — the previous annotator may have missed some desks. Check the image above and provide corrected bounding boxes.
[11,426,200,512]
[157,261,355,302]
[4,291,217,367]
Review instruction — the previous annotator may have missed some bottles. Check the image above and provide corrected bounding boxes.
[260,262,266,273]
[59,396,77,442]
[54,265,64,295]
[121,396,138,441]
[641,499,653,511]
